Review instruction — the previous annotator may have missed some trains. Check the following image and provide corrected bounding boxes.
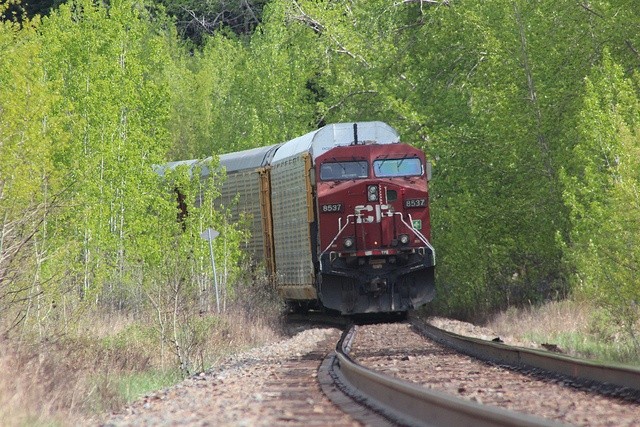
[147,118,438,320]
